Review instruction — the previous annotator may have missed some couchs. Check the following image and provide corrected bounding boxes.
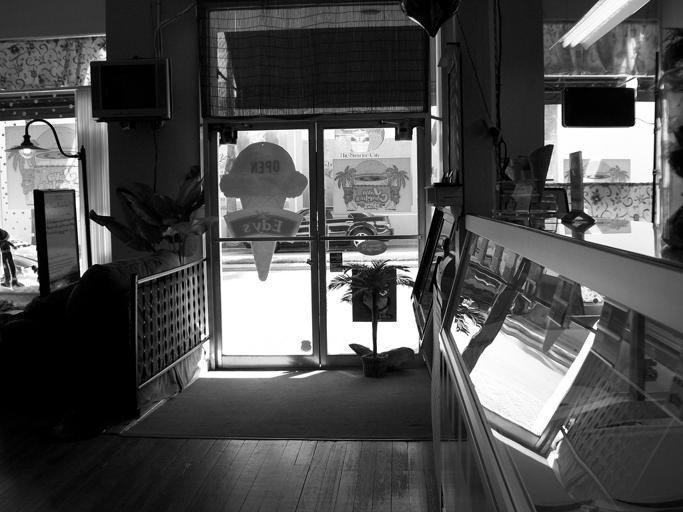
[0,249,204,425]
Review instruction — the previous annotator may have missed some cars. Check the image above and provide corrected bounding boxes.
[242,206,395,251]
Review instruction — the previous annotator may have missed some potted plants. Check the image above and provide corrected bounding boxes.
[327,258,417,378]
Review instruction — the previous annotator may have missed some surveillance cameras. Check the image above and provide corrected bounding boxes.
[219,128,236,144]
[395,125,413,139]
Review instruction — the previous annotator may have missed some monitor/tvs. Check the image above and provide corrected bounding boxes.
[91,60,166,118]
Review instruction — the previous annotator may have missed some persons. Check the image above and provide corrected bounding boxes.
[0,228,24,288]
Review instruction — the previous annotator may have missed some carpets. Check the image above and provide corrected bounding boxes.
[118,370,444,441]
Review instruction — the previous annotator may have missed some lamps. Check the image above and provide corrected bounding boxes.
[550,0,649,51]
[5,116,93,273]
